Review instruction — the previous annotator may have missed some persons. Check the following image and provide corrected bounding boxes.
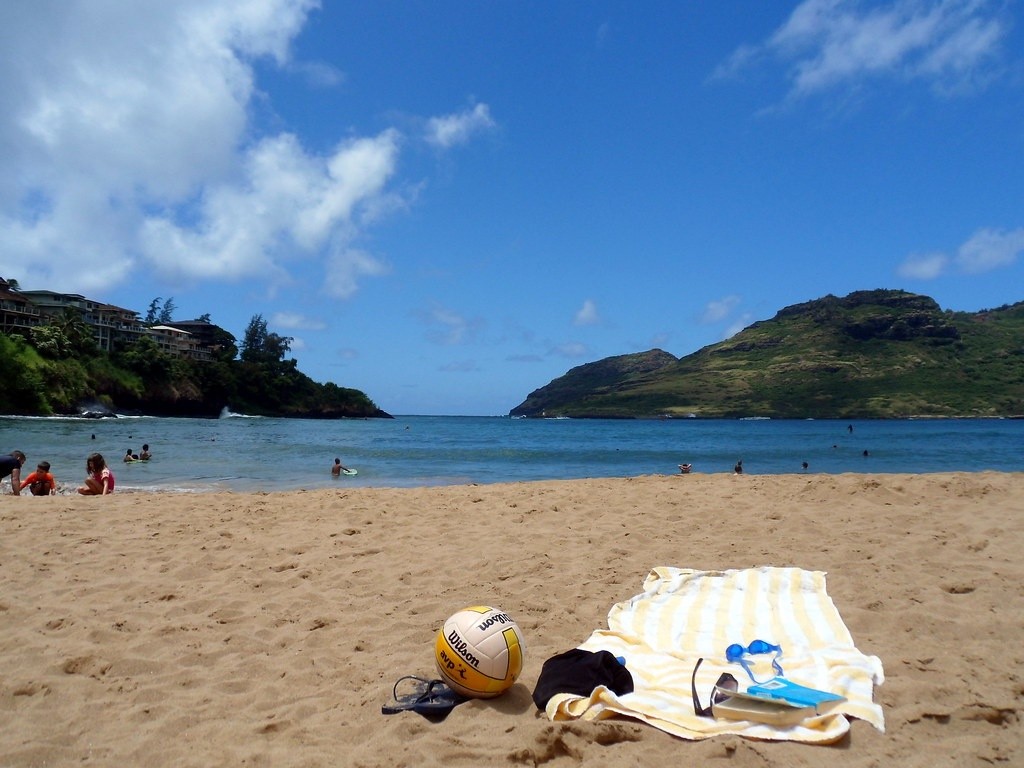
[139,444,149,460]
[864,450,868,456]
[79,453,114,495]
[848,424,853,432]
[735,460,743,474]
[331,458,350,476]
[802,462,809,469]
[123,448,138,462]
[19,462,55,496]
[0,450,27,496]
[678,464,692,473]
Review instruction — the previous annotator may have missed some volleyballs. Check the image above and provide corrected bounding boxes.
[435,605,528,697]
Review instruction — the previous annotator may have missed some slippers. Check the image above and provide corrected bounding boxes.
[381,675,468,714]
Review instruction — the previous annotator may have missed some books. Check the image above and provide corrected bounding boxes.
[711,677,848,726]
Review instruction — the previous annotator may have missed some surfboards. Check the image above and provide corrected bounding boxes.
[343,469,357,476]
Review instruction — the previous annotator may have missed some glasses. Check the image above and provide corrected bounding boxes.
[726,640,777,663]
[691,657,738,716]
